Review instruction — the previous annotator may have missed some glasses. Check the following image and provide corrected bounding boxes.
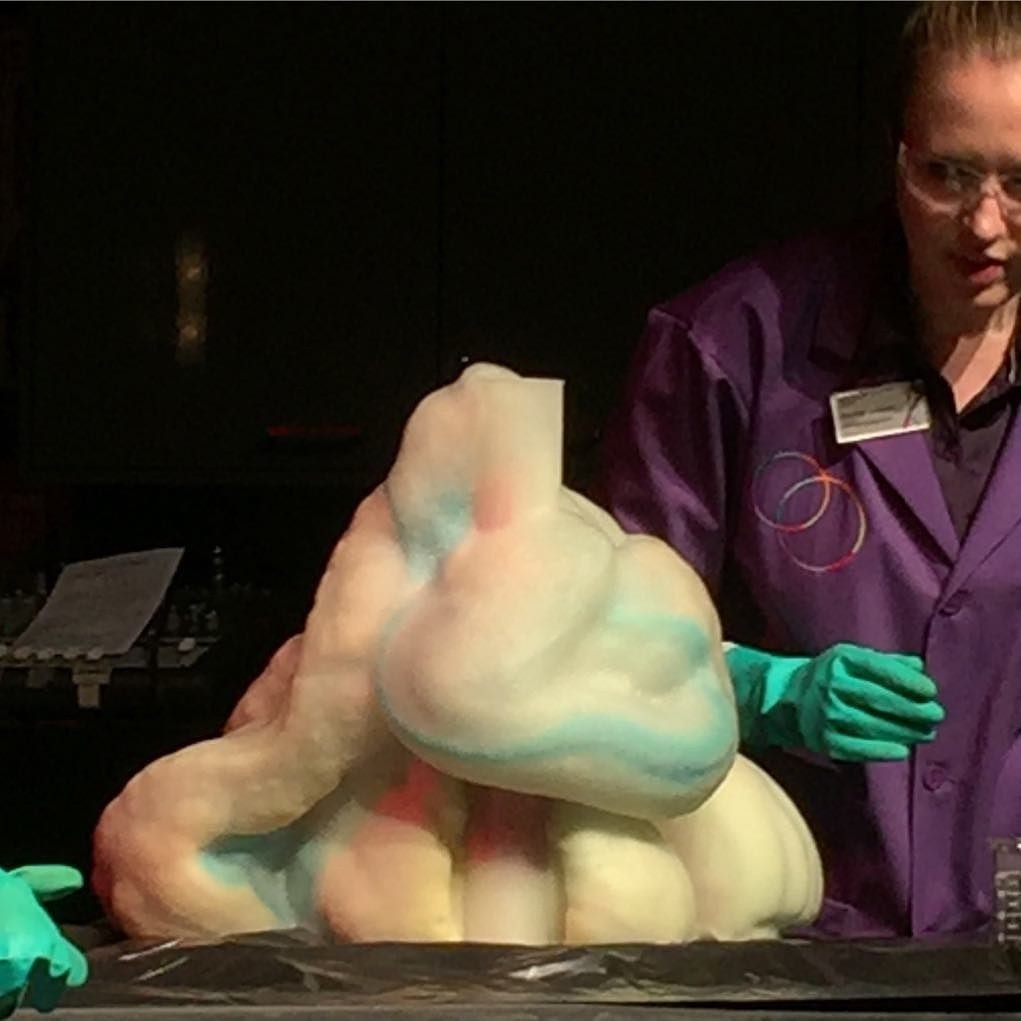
[898,142,1021,205]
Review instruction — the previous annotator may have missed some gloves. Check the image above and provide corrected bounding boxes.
[720,644,944,763]
[0,864,88,1014]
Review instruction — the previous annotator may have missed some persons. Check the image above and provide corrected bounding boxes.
[585,2,1021,944]
[0,856,95,1014]
